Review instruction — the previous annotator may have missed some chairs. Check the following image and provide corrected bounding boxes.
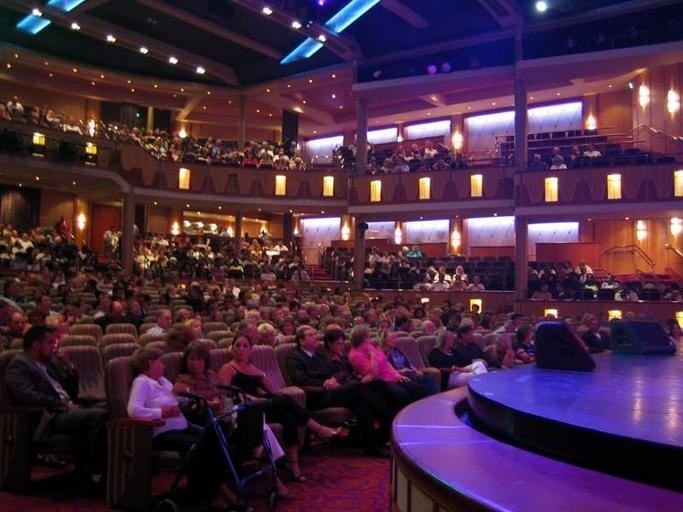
[0,275,314,511]
[335,127,678,174]
[315,250,658,425]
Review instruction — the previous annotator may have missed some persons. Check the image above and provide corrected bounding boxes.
[0,95,306,171]
[175,346,297,500]
[2,324,106,500]
[122,345,256,511]
[528,143,600,171]
[332,135,452,175]
[0,211,683,458]
[217,331,345,482]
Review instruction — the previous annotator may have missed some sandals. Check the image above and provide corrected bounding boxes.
[277,489,302,501]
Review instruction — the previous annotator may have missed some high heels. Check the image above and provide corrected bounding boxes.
[321,425,352,445]
[286,463,307,482]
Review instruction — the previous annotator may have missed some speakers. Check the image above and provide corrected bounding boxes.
[609,318,676,355]
[535,322,596,372]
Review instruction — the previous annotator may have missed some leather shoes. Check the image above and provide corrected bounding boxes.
[364,445,390,458]
